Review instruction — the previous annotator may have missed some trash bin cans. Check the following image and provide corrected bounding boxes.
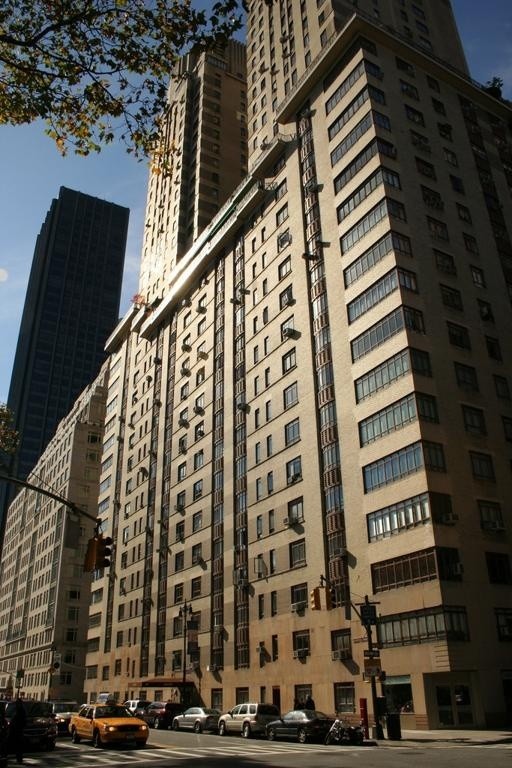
[386,714,401,741]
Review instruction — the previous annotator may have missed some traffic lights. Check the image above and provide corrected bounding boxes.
[326,586,339,608]
[310,587,320,610]
[95,534,113,570]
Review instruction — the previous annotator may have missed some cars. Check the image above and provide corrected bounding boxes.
[398,698,414,712]
[454,690,465,702]
[0,691,183,750]
[264,707,334,743]
[172,706,222,733]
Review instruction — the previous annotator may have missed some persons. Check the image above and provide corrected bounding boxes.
[293,698,303,711]
[4,699,27,764]
[305,692,318,710]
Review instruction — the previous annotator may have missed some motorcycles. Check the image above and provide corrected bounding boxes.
[324,711,366,745]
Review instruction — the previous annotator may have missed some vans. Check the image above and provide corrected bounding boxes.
[217,701,282,738]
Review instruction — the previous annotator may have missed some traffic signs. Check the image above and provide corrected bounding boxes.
[364,650,380,656]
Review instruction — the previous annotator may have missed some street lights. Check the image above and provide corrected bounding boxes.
[178,597,193,706]
